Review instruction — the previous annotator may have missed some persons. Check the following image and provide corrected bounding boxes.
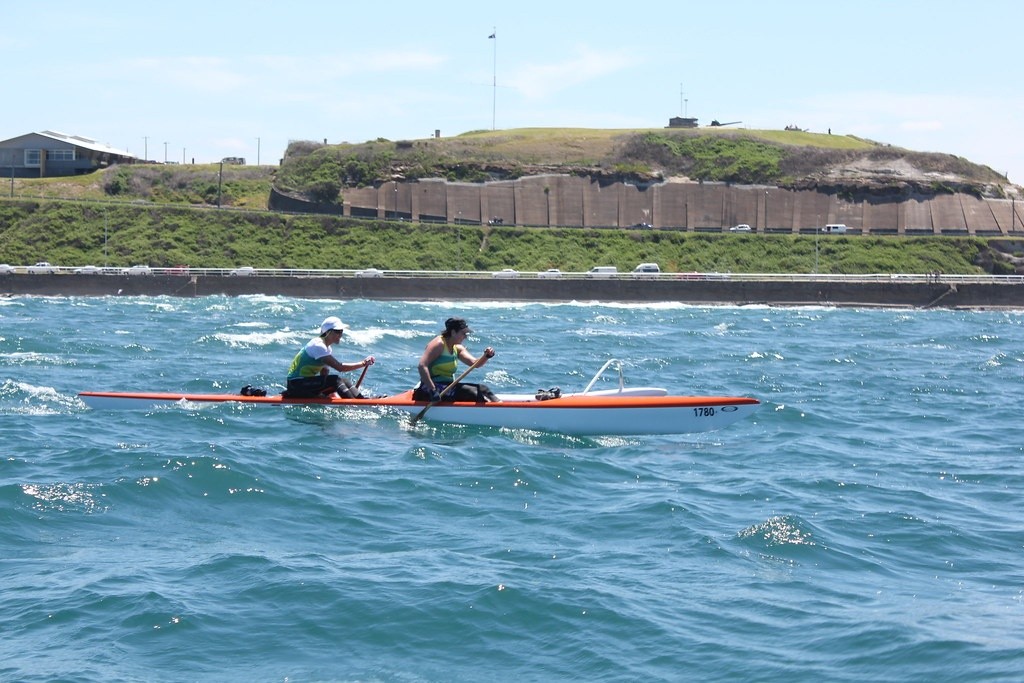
[286,315,375,399]
[418,317,504,405]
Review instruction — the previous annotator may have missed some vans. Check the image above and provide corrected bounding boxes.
[585,266,618,279]
[825,224,846,234]
[632,261,660,278]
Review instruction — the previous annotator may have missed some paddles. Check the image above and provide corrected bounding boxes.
[355,364,370,390]
[408,349,495,427]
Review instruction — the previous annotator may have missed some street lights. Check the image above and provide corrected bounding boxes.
[102,207,109,268]
[457,211,462,272]
[815,214,821,276]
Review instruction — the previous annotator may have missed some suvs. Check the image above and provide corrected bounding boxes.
[538,269,563,278]
[229,267,256,277]
[729,224,752,233]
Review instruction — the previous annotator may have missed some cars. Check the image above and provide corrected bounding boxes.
[354,268,385,278]
[491,268,521,279]
[27,262,61,275]
[0,264,16,275]
[122,156,247,165]
[121,264,153,276]
[626,222,655,230]
[73,265,104,276]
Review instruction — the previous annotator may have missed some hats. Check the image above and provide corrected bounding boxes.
[321,316,349,334]
[448,323,473,333]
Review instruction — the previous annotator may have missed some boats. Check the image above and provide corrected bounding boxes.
[76,357,761,436]
